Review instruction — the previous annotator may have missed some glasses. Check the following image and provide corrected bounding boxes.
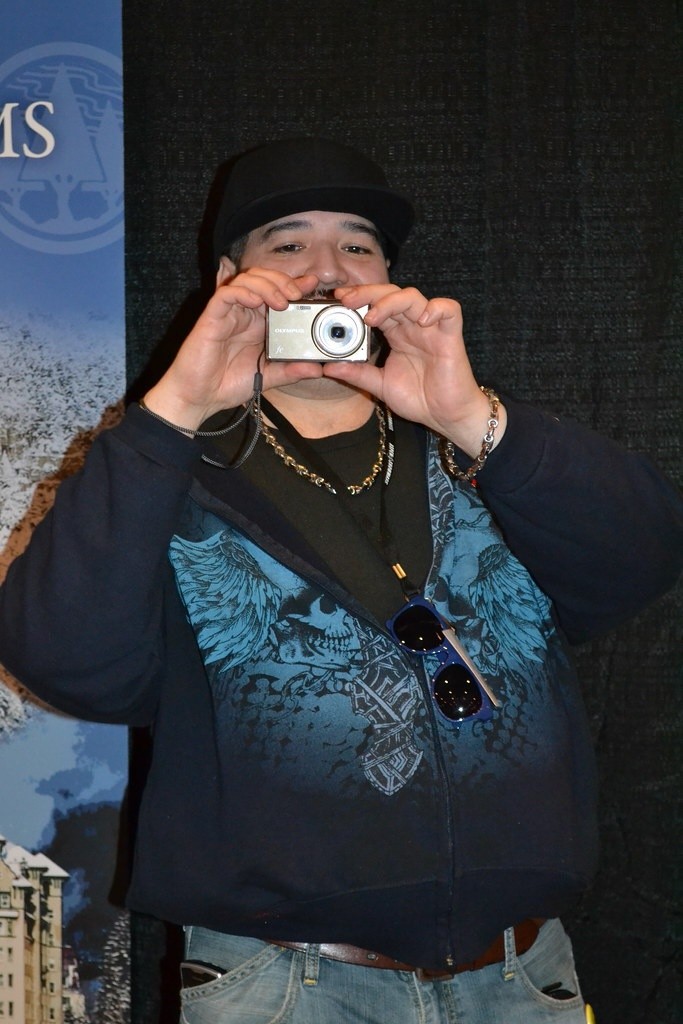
[386,596,501,731]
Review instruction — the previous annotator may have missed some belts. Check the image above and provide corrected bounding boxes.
[269,917,547,982]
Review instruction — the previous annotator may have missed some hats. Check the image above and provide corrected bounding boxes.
[198,135,415,270]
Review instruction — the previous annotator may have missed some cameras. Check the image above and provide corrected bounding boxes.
[265,299,371,363]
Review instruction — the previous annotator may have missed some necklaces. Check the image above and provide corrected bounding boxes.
[246,396,387,495]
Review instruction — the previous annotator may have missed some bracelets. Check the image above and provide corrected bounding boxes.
[445,386,499,482]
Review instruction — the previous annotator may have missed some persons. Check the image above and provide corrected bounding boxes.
[0,139,683,1024]
